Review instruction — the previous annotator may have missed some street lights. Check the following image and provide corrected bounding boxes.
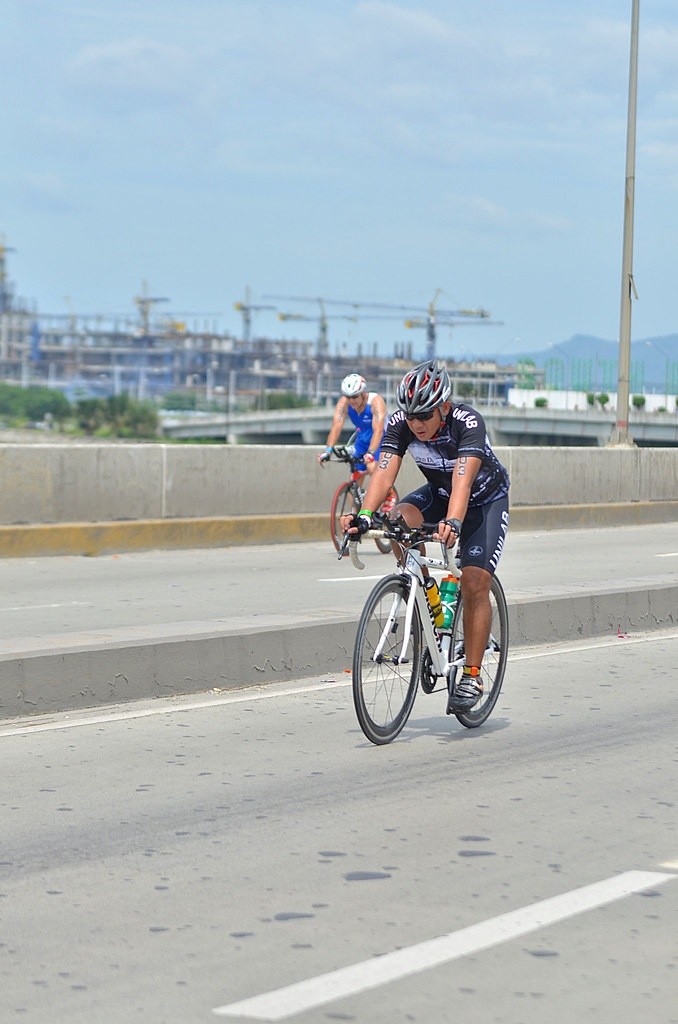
[646,340,670,409]
[547,340,569,358]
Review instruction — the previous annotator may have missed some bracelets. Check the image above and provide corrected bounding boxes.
[325,447,333,452]
[357,510,372,519]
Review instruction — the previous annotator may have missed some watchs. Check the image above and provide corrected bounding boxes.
[450,518,462,534]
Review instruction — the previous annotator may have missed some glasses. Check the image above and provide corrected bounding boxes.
[344,395,360,399]
[405,409,435,421]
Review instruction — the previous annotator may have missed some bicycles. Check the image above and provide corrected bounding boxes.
[318,446,400,555]
[338,523,511,746]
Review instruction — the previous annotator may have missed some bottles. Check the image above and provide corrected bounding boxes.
[423,576,444,627]
[439,574,458,629]
[361,489,367,498]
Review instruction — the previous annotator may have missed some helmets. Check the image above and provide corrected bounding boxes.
[341,373,366,397]
[395,359,451,414]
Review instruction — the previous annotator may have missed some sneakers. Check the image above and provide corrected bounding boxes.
[449,673,484,709]
[381,489,397,512]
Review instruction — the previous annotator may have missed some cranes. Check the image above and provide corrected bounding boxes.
[275,287,507,356]
[135,281,171,337]
[235,284,278,342]
[0,236,18,314]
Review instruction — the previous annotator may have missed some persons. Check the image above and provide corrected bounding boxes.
[340,359,510,710]
[317,373,396,513]
